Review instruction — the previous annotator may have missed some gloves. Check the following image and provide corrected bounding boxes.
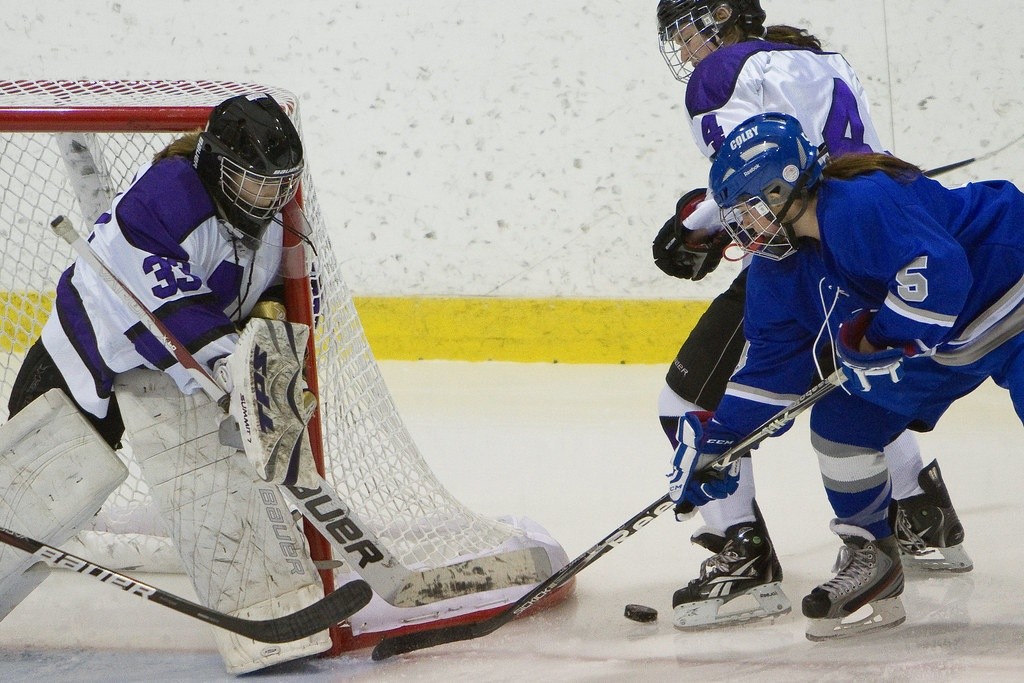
[835,308,906,393]
[667,411,742,520]
[653,188,737,281]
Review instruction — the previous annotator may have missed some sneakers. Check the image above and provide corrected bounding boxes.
[671,497,790,630]
[801,497,906,642]
[889,458,975,573]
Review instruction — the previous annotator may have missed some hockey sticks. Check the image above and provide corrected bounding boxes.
[1,529,373,644]
[49,214,555,609]
[370,366,848,662]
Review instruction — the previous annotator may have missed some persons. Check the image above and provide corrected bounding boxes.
[653,0,974,630]
[0,92,334,676]
[668,112,1024,642]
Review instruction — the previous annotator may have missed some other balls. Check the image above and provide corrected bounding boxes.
[624,604,658,623]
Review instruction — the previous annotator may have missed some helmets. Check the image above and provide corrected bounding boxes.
[655,0,767,86]
[709,111,827,262]
[190,92,305,250]
